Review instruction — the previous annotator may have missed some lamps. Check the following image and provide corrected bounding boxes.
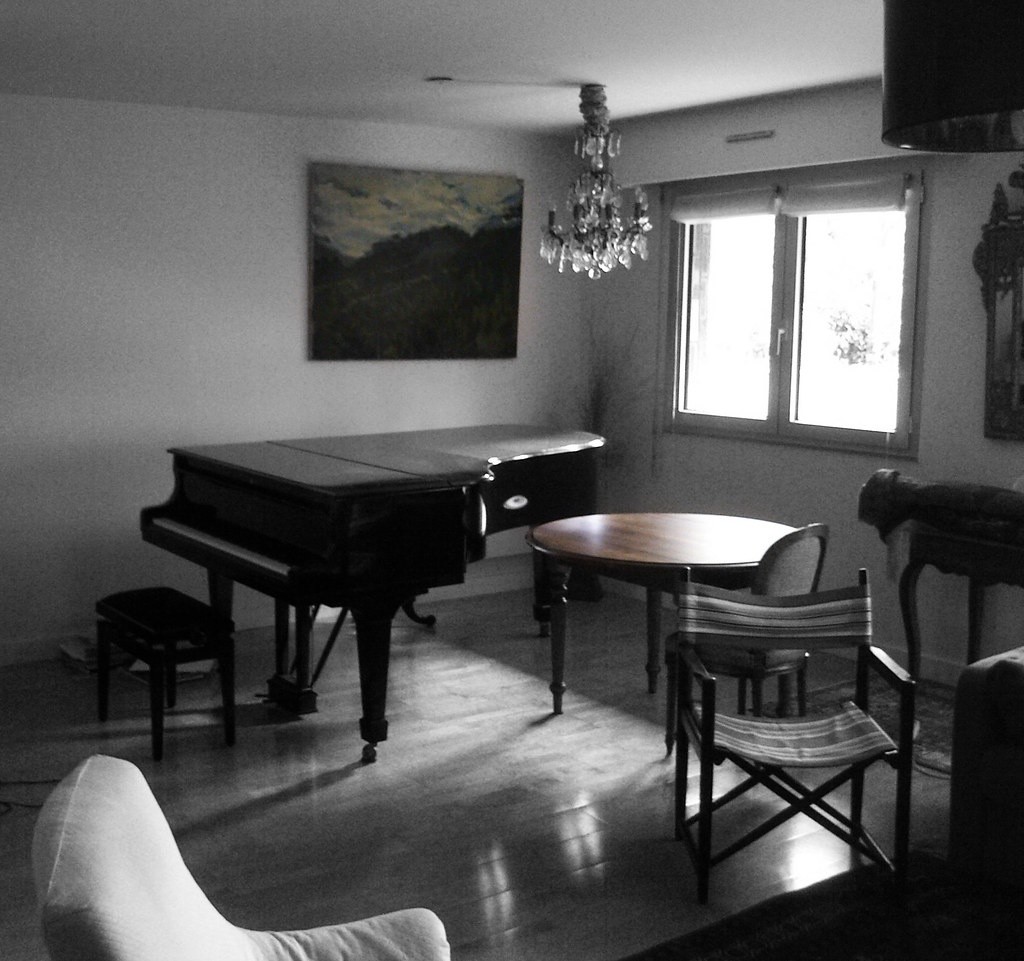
[540,82,655,281]
[973,182,1023,442]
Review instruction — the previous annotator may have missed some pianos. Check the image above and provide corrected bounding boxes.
[138,422,607,765]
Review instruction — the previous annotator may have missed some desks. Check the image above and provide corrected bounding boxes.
[524,512,797,751]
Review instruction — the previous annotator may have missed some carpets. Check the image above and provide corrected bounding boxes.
[620,845,1024,961]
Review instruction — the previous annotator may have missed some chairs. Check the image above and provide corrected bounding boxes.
[665,521,829,772]
[669,566,915,906]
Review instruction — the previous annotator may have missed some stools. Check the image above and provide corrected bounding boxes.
[97,586,238,758]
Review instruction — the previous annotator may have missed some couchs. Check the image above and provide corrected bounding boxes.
[30,753,453,961]
[948,644,1024,879]
